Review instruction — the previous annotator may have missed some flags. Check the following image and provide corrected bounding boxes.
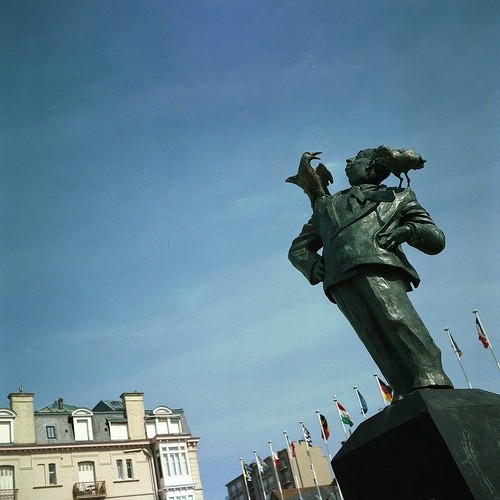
[475,316,489,350]
[357,390,368,414]
[377,377,393,403]
[286,436,296,458]
[337,401,353,427]
[242,460,252,482]
[272,451,280,466]
[450,333,463,358]
[256,454,268,474]
[302,425,312,447]
[319,414,330,441]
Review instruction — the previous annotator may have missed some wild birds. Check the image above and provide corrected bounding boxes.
[374,145,426,188]
[284,152,334,208]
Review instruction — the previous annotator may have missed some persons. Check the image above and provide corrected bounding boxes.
[287,148,456,392]
